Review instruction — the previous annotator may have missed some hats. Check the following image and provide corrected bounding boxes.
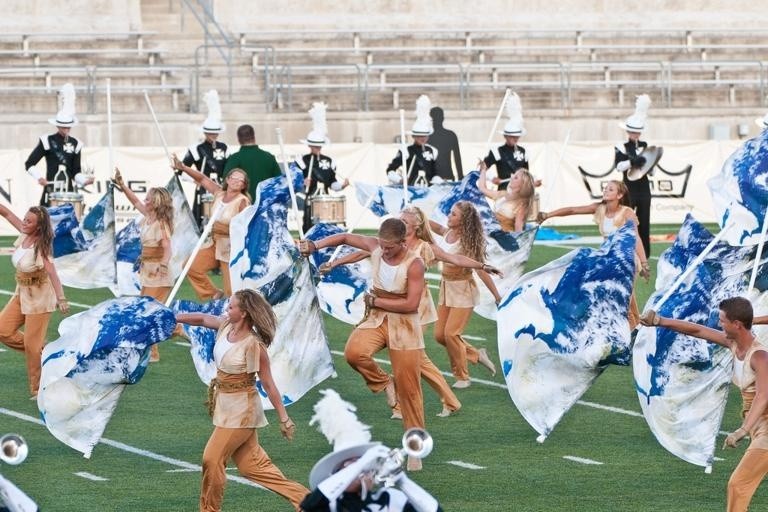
[202,89,226,134]
[625,93,651,133]
[306,102,328,147]
[308,387,384,493]
[48,82,78,128]
[502,89,525,137]
[411,94,434,136]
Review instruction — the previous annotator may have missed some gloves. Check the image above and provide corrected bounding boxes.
[317,443,390,502]
[0,474,37,512]
[397,475,438,512]
[631,156,646,167]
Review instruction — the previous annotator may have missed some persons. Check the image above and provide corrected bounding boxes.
[0,86,767,512]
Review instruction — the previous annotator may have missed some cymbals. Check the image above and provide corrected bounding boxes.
[627,146,663,181]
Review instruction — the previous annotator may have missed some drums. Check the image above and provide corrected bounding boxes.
[202,193,213,216]
[525,194,540,222]
[310,197,345,223]
[51,192,83,219]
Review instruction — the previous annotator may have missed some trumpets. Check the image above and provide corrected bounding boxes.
[361,428,432,485]
